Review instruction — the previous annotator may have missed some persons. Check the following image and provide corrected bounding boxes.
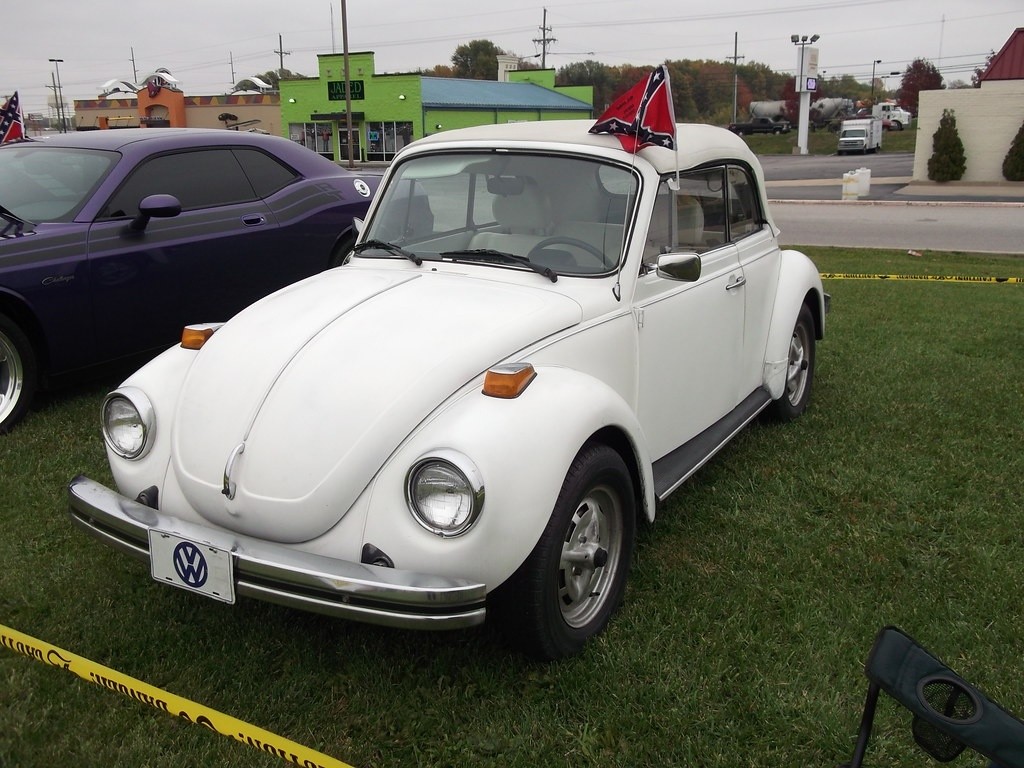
[300,128,330,152]
[402,126,410,146]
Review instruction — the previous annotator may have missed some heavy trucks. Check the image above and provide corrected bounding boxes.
[749,98,912,132]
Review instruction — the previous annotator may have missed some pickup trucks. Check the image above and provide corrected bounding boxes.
[728,117,792,137]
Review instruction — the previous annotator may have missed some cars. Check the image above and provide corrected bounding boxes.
[68,61,833,670]
[1,125,438,441]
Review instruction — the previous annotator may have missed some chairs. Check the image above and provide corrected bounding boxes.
[641,194,703,267]
[468,176,556,257]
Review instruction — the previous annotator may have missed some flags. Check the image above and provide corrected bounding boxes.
[0,90,26,145]
[588,64,679,156]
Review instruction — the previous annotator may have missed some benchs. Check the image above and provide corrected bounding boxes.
[544,219,741,267]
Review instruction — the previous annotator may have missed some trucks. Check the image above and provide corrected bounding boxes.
[835,119,883,154]
